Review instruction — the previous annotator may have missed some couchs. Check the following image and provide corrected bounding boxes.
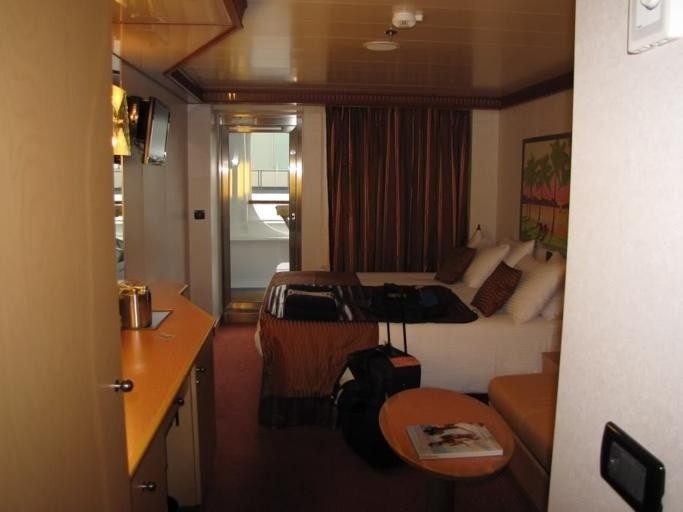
[487,351,560,512]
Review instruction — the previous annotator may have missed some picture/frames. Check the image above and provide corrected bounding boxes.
[519,132,572,265]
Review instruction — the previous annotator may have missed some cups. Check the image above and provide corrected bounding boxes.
[122,288,152,331]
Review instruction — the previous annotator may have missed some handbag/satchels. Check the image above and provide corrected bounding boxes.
[284,284,337,320]
[366,283,421,318]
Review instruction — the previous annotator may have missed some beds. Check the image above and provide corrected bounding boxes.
[255,271,563,431]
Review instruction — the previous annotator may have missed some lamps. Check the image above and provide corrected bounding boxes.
[364,41,401,52]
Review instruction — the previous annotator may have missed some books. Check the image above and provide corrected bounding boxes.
[406,422,504,460]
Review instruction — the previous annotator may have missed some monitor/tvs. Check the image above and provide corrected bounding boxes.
[142,95,172,166]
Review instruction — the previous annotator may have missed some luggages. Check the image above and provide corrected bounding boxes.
[333,282,421,470]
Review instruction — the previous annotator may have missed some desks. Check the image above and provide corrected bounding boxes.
[120,280,219,512]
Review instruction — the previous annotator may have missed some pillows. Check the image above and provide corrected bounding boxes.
[433,236,566,325]
